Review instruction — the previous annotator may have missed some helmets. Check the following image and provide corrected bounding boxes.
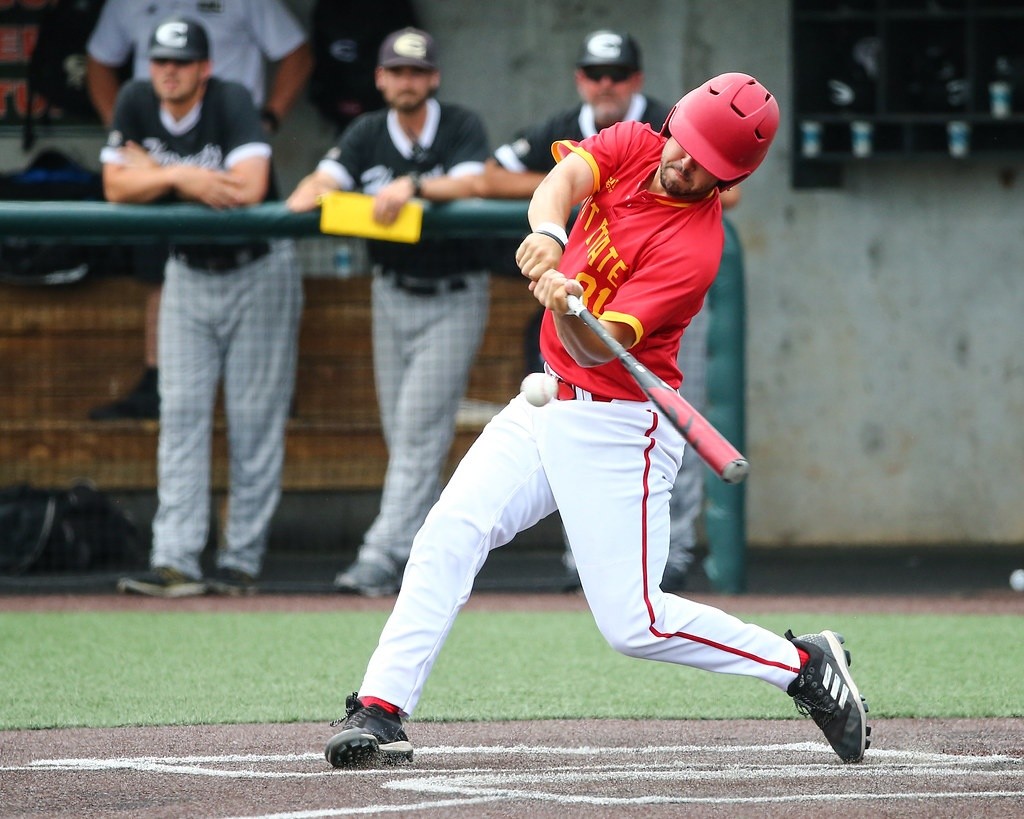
[659,72,780,194]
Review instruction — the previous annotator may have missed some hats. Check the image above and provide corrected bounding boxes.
[576,29,640,73]
[379,27,438,72]
[149,18,208,61]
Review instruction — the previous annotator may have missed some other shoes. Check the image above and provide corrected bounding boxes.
[659,566,685,593]
[117,566,207,598]
[208,568,262,597]
[333,543,402,598]
[562,574,581,594]
[88,367,159,420]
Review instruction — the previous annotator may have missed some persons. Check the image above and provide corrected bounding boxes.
[288,25,495,598]
[97,17,306,597]
[483,30,710,596]
[80,0,318,422]
[323,71,874,772]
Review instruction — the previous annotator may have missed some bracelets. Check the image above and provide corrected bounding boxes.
[409,171,423,200]
[533,229,566,254]
[263,105,284,133]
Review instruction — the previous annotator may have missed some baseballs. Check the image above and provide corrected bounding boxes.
[519,373,559,407]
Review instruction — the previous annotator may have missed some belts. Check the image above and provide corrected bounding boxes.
[382,267,465,297]
[171,242,271,274]
[546,363,612,402]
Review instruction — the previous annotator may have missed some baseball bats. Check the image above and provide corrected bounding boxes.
[566,291,751,488]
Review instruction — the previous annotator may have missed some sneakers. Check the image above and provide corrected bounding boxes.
[784,629,871,764]
[325,691,413,769]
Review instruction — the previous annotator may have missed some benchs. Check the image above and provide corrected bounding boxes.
[0,270,548,575]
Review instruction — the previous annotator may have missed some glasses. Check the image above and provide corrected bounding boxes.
[580,67,636,81]
